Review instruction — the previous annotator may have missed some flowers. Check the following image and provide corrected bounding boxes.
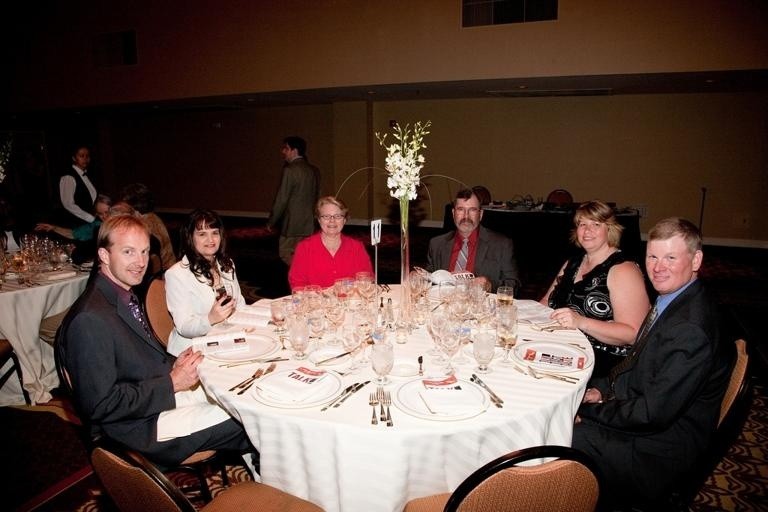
[376,121,433,247]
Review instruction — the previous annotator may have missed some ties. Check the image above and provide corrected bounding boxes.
[608,305,657,382]
[129,299,151,339]
[454,232,469,272]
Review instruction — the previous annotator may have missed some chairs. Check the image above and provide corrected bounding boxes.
[146,279,176,348]
[663,338,756,512]
[89,439,325,512]
[403,445,609,512]
[53,327,228,506]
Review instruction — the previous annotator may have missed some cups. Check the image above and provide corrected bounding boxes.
[500,306,518,334]
[454,278,472,301]
[357,272,371,295]
[304,285,322,309]
[308,312,325,351]
[271,301,287,334]
[326,306,345,346]
[454,317,471,363]
[498,287,513,313]
[290,319,309,360]
[497,320,519,364]
[341,324,361,370]
[342,279,354,313]
[441,323,460,370]
[371,342,394,385]
[293,287,308,314]
[334,283,347,303]
[431,312,450,344]
[353,313,374,367]
[439,280,457,316]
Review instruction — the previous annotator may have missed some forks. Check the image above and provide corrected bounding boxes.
[513,364,580,384]
[237,363,277,395]
[240,369,264,388]
[382,391,394,427]
[369,393,379,425]
[376,386,387,421]
[229,369,260,391]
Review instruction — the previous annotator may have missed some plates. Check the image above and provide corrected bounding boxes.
[388,360,426,377]
[510,341,593,373]
[464,343,504,360]
[429,284,455,301]
[391,375,490,421]
[204,332,280,363]
[251,366,346,409]
[308,345,350,365]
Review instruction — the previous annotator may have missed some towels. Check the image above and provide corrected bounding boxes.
[44,269,77,281]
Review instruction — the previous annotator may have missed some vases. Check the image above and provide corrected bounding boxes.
[399,226,413,335]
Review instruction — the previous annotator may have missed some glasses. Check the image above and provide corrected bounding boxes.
[320,213,345,220]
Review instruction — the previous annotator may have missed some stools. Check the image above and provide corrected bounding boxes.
[0,339,32,406]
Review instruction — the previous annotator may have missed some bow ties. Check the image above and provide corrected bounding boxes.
[82,172,88,176]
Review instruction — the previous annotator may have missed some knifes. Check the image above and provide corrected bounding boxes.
[315,351,351,365]
[321,382,359,411]
[219,357,290,368]
[334,381,371,409]
[472,374,504,403]
[418,356,424,376]
[470,377,503,408]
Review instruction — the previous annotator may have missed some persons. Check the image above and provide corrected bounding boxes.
[58,146,103,224]
[164,207,246,360]
[38,203,161,345]
[35,190,114,241]
[424,190,520,294]
[571,217,727,480]
[120,182,177,274]
[289,195,375,293]
[265,136,321,266]
[64,216,261,474]
[538,198,650,388]
